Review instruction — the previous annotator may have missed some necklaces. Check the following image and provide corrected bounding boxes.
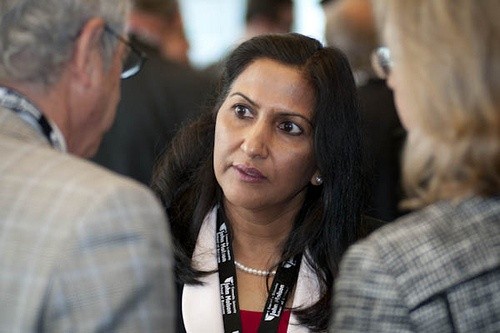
[234,261,276,277]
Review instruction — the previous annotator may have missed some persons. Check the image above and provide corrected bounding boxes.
[0,0,500,333]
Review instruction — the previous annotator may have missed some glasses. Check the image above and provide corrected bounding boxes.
[105,23,148,79]
[371,44,396,79]
